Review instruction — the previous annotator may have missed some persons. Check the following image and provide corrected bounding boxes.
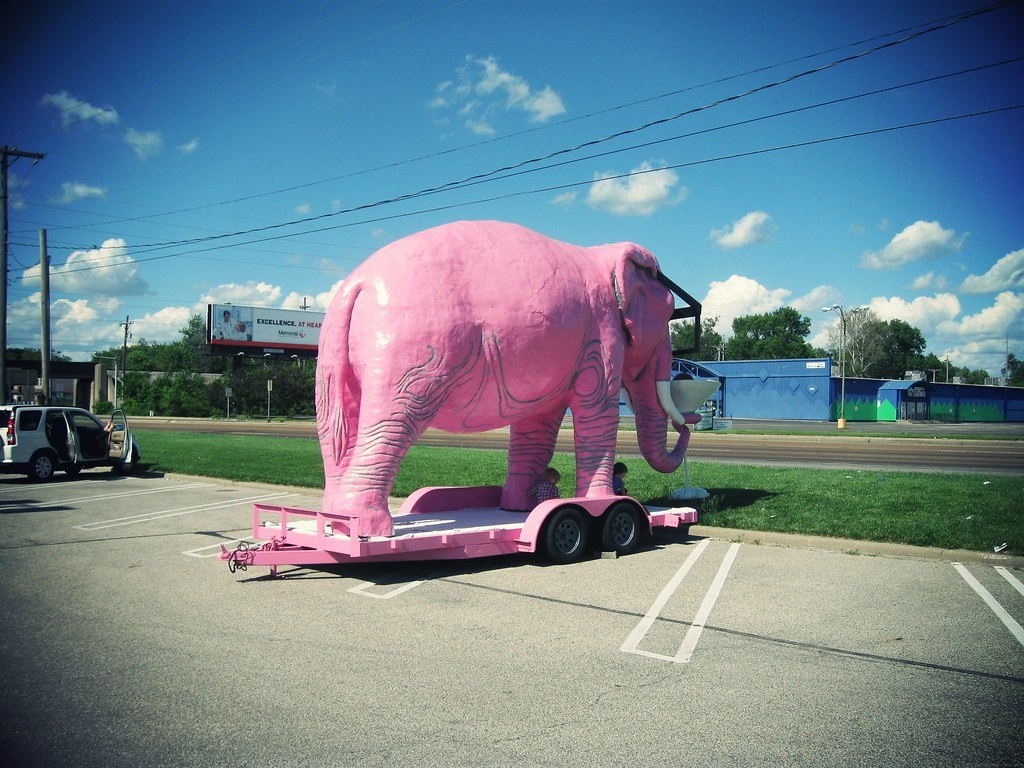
[80,421,114,446]
[528,468,561,505]
[221,310,247,341]
[612,462,628,496]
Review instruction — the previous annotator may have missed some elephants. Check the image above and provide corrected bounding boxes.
[314,218,703,538]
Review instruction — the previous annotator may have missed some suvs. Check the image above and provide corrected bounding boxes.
[0,405,141,480]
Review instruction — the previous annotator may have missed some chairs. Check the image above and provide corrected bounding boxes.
[50,416,67,447]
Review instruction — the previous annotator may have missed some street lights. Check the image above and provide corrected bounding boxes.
[821,304,871,427]
